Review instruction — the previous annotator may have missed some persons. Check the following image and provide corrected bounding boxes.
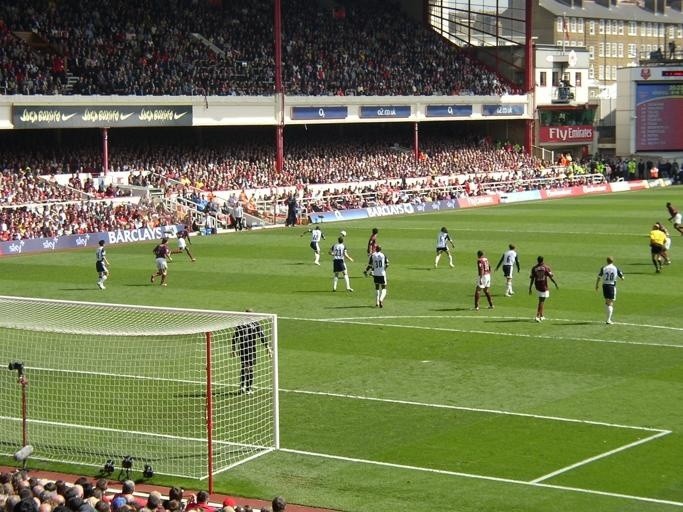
[1,1,524,96]
[649,222,671,274]
[151,237,172,286]
[96,240,110,289]
[0,466,287,512]
[301,226,325,265]
[665,202,681,232]
[364,245,389,308]
[0,125,681,240]
[434,227,456,268]
[231,308,272,394]
[528,256,558,322]
[328,231,354,292]
[364,228,379,277]
[171,226,195,261]
[496,244,521,298]
[596,256,624,323]
[472,250,495,310]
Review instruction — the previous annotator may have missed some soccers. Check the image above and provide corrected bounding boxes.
[339,230,346,237]
